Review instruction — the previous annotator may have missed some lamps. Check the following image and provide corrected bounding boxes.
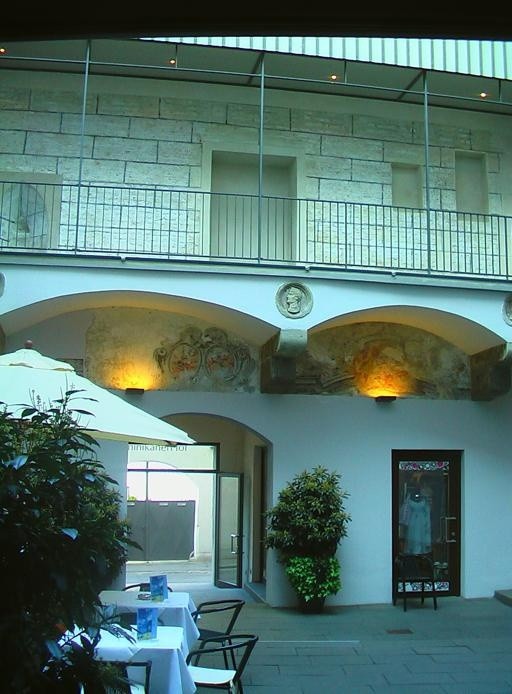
[376,393,395,402]
[125,387,144,392]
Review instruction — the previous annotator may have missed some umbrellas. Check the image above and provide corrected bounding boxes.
[0,340,195,447]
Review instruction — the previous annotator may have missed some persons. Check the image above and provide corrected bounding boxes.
[286,287,303,313]
[400,489,431,555]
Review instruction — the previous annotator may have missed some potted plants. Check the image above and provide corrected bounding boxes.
[259,462,354,614]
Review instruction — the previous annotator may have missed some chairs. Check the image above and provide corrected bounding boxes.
[100,611,165,626]
[192,597,245,670]
[91,657,152,694]
[186,632,260,693]
[123,584,173,592]
[393,553,439,611]
